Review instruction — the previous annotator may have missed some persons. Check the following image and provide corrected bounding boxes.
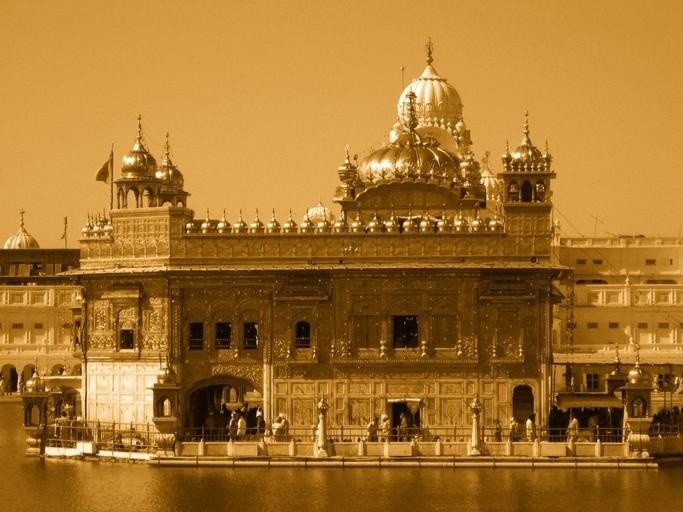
[587,414,600,430]
[566,412,580,442]
[526,414,535,442]
[0,376,12,396]
[57,412,84,441]
[509,417,521,441]
[271,413,289,442]
[205,403,263,441]
[367,414,391,442]
[399,413,408,436]
[495,419,502,442]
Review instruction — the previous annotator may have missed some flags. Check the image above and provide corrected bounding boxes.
[95,150,112,183]
